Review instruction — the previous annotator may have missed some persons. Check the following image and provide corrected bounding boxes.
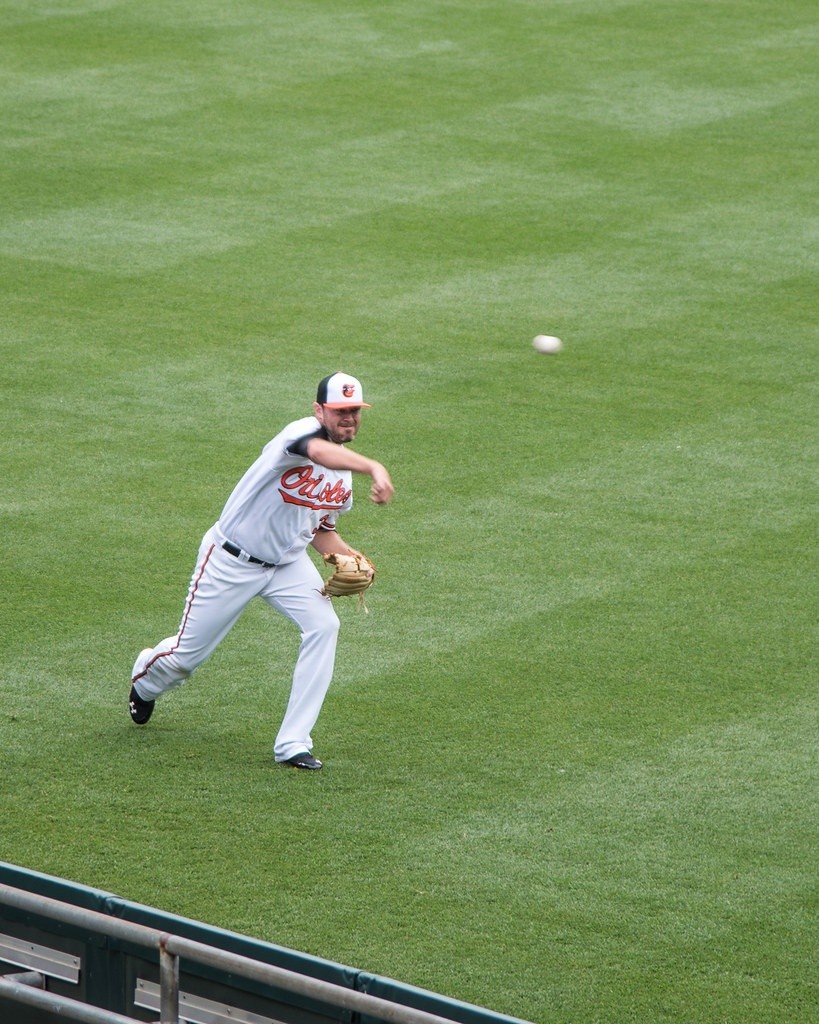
[129,372,395,770]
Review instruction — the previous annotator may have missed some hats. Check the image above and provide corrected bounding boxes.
[317,371,371,408]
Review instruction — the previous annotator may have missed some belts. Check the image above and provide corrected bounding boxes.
[223,541,275,568]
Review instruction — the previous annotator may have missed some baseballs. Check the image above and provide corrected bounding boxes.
[535,336,561,354]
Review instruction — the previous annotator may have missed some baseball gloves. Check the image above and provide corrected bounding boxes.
[320,551,376,598]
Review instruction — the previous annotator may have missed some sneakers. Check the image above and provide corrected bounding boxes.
[285,752,322,770]
[130,648,155,724]
